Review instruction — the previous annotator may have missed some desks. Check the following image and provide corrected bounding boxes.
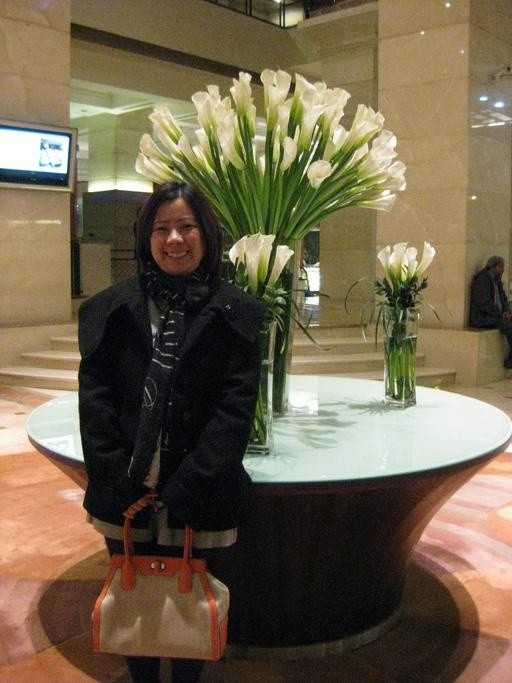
[26,373,512,663]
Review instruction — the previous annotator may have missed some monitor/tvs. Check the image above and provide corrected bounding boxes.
[0,119,78,194]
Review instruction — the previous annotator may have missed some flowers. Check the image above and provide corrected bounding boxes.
[135,67,407,405]
[344,240,444,400]
[227,234,320,442]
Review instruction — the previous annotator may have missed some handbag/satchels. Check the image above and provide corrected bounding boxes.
[87,497,235,663]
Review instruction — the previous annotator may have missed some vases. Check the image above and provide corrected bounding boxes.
[382,304,421,408]
[242,319,281,457]
[273,241,302,418]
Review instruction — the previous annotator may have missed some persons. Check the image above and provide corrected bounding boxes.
[466,255,511,369]
[77,182,267,682]
